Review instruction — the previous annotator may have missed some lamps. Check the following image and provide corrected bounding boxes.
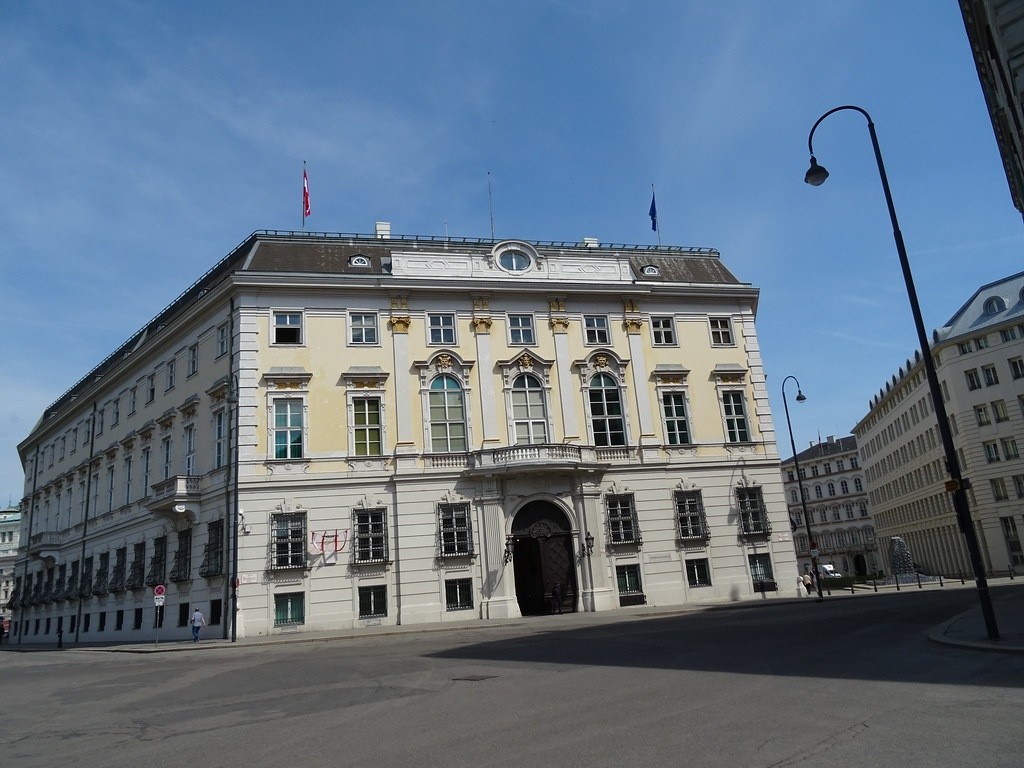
[582,531,594,557]
[504,536,514,563]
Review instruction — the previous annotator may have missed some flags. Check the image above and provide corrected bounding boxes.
[303,168,311,217]
[649,191,658,232]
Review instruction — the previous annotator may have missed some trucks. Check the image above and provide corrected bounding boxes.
[807,564,842,582]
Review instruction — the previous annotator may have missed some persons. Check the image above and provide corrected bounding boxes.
[810,566,825,596]
[438,355,454,367]
[190,607,206,642]
[519,356,534,368]
[596,356,609,367]
[803,572,812,595]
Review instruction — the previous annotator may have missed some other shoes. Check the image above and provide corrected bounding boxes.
[194,640,196,643]
[197,637,200,642]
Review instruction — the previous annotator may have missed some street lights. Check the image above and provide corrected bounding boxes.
[225,372,240,642]
[803,104,1002,639]
[780,375,825,597]
